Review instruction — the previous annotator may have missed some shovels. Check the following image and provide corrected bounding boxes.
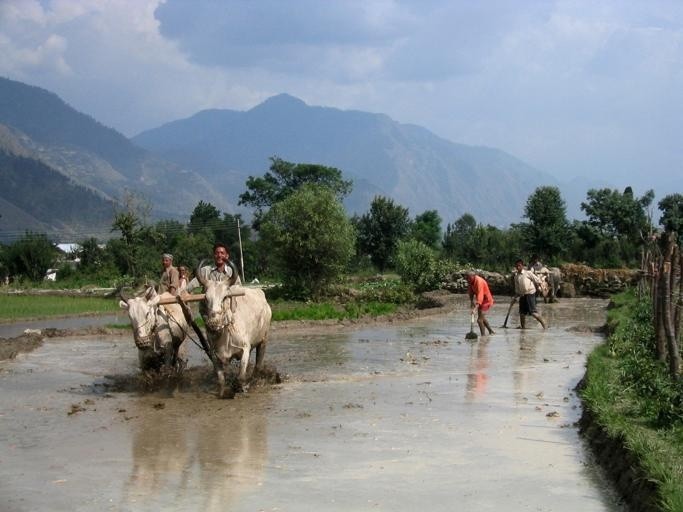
[466,304,477,339]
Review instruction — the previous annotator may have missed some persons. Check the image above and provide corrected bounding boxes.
[175,264,188,294]
[458,269,495,336]
[157,252,179,295]
[508,258,551,331]
[464,335,491,407]
[512,332,547,402]
[186,243,241,345]
[527,254,543,271]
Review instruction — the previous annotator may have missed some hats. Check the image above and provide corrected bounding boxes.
[162,253,174,261]
[465,271,476,276]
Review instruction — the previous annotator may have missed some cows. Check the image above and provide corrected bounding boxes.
[196,257,272,399]
[539,267,560,304]
[119,287,192,390]
